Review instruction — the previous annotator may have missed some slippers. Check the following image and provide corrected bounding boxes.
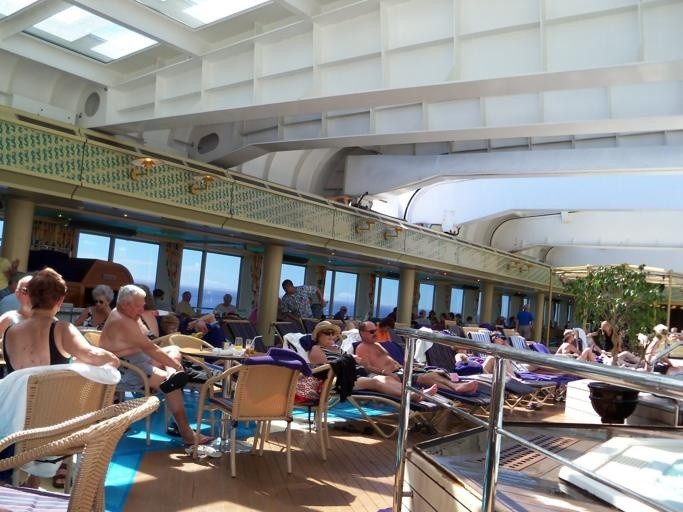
[159,371,189,394]
[186,435,214,445]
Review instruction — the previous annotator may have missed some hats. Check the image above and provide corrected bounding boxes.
[311,321,341,341]
[563,330,574,340]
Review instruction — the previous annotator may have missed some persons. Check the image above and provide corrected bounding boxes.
[497,304,534,338]
[0,272,257,368]
[310,319,479,404]
[411,311,474,331]
[490,331,539,372]
[281,279,326,322]
[557,320,683,377]
[388,307,397,322]
[99,284,214,445]
[333,306,347,321]
[2,266,121,488]
[451,346,495,375]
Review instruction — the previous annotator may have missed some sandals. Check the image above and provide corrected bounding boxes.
[54,463,72,488]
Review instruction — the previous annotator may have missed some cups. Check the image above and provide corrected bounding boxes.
[235,337,243,351]
[223,342,230,350]
[245,339,255,354]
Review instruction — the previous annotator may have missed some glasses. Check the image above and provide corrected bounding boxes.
[322,332,335,335]
[365,330,376,334]
[94,300,103,304]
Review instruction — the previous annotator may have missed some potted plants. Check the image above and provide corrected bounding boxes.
[564,264,668,423]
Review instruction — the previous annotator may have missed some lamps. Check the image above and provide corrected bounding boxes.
[130,157,165,181]
[190,174,216,194]
[506,260,535,273]
[356,219,379,233]
[384,226,403,240]
